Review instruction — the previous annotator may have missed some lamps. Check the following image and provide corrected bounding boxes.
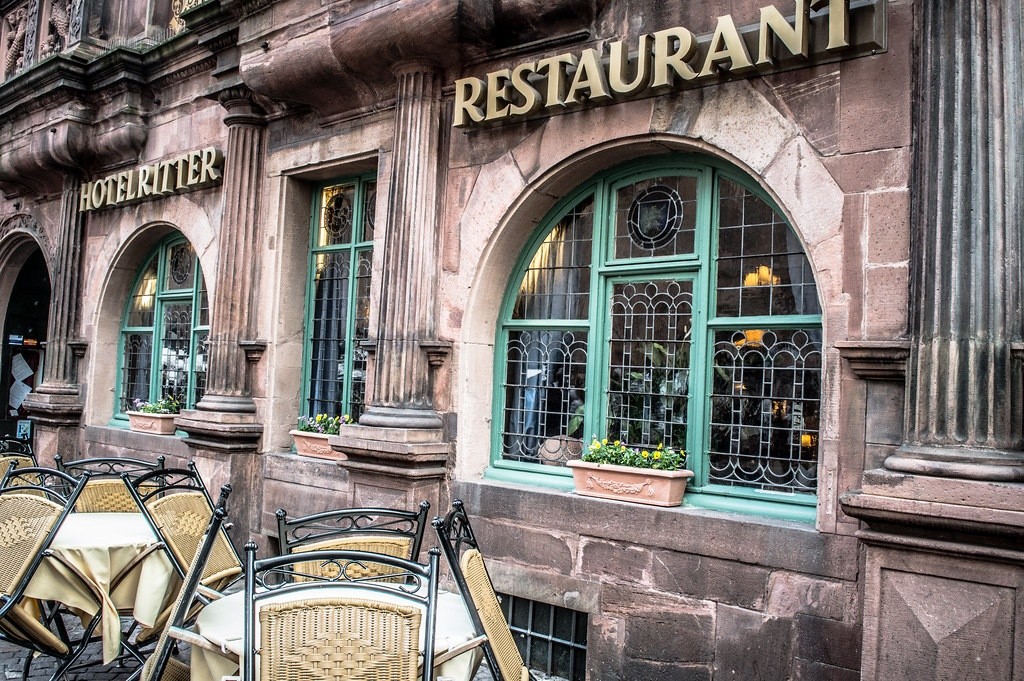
[735,330,765,349]
[802,433,812,449]
[744,264,781,289]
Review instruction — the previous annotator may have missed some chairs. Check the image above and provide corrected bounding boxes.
[0,433,536,681]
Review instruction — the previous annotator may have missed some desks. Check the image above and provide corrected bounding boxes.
[190,582,485,681]
[20,513,184,681]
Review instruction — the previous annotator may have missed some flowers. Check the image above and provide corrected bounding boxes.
[582,434,685,471]
[133,394,180,415]
[297,413,359,435]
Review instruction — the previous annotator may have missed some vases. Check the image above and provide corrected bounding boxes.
[290,430,348,461]
[565,460,695,507]
[125,411,180,435]
